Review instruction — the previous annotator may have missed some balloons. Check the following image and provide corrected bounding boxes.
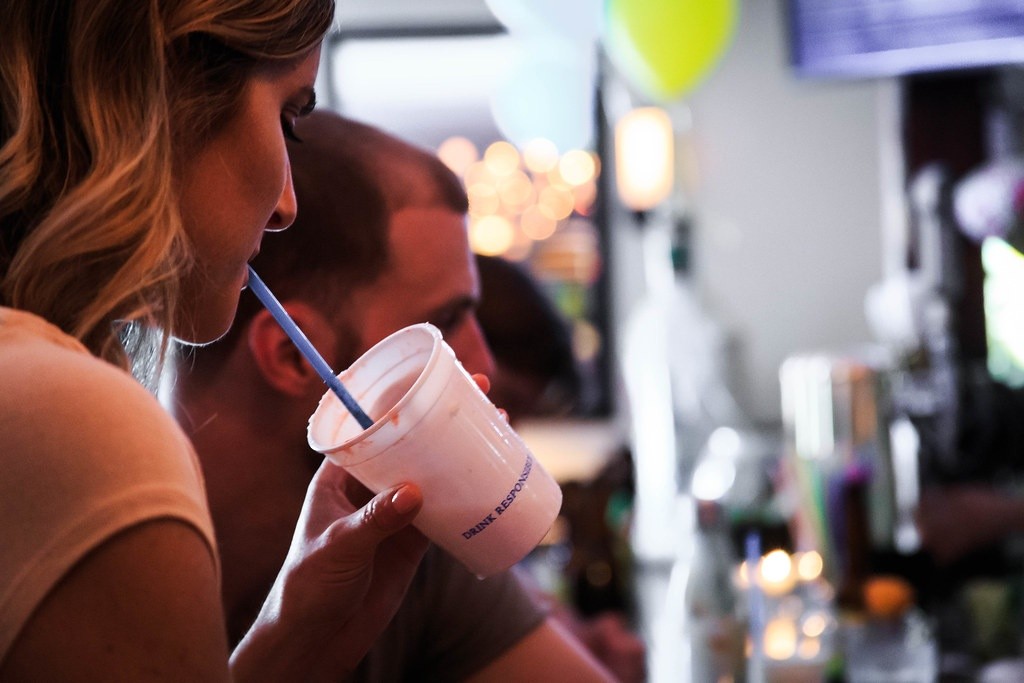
[479,0,738,155]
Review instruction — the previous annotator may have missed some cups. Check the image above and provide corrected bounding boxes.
[306,323,562,576]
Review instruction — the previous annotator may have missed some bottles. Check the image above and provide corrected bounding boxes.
[666,499,749,683]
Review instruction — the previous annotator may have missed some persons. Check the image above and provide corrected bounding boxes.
[1,0,508,682]
[155,108,622,682]
[472,254,753,682]
